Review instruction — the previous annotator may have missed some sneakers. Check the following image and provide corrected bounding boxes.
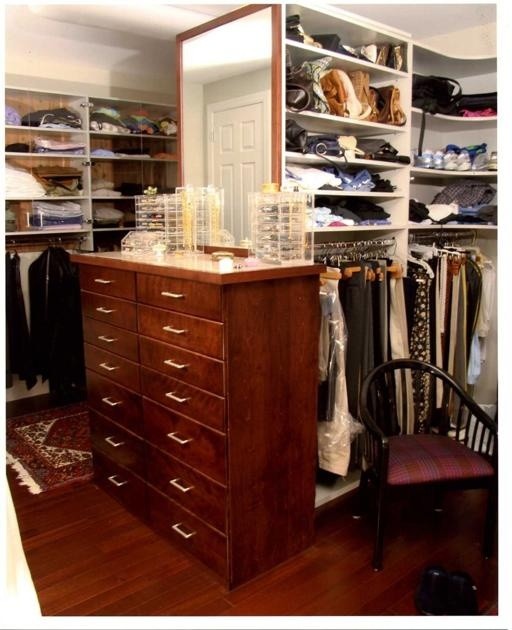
[415,142,497,172]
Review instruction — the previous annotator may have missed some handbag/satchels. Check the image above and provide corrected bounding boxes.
[413,72,461,116]
[283,15,411,161]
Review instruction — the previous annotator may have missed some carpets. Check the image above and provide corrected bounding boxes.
[7,402,95,497]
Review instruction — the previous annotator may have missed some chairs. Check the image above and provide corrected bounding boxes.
[359,360,497,574]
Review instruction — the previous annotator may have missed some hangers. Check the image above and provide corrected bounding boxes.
[41,237,65,278]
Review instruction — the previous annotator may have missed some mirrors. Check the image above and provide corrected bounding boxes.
[175,3,285,256]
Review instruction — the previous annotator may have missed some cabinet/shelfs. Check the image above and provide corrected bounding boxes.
[5,87,180,417]
[283,5,498,517]
[68,250,327,593]
